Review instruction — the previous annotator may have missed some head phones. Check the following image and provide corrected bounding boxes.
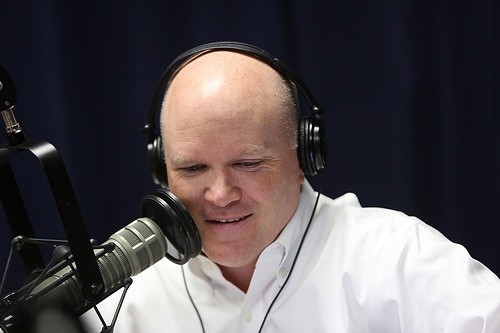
[145,41,325,192]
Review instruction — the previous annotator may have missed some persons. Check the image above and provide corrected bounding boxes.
[81,42,500,333]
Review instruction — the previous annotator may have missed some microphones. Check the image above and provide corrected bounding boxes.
[0,217,168,333]
